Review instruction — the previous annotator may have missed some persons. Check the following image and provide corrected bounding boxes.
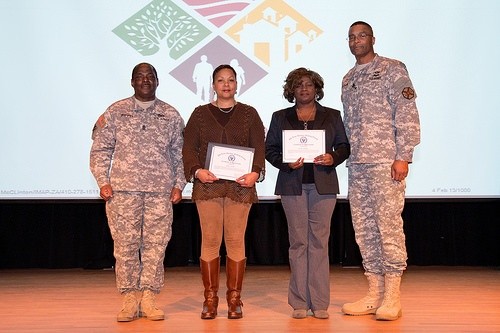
[341,21,421,322]
[264,68,351,319]
[89,63,187,322]
[182,65,266,319]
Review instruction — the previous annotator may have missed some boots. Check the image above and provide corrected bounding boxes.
[199,257,219,323]
[225,254,247,319]
[341,274,384,316]
[375,275,403,320]
[138,290,165,320]
[116,292,139,322]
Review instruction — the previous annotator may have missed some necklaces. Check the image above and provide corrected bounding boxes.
[215,100,235,113]
[297,104,315,130]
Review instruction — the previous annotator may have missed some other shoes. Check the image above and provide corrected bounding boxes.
[313,310,329,319]
[292,309,307,318]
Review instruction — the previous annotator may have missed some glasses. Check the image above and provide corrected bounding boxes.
[345,32,372,42]
